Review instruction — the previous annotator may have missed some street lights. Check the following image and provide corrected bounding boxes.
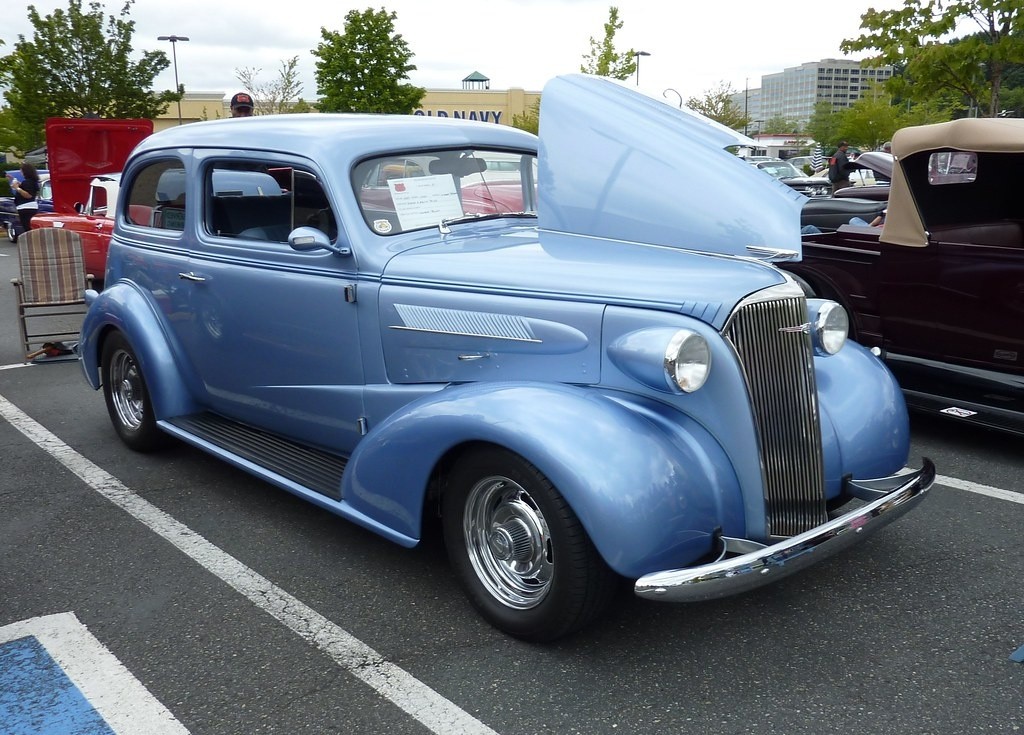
[633,51,651,87]
[157,35,190,125]
[754,120,764,134]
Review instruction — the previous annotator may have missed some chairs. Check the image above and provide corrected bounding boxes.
[9,227,95,365]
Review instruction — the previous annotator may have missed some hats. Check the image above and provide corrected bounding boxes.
[837,141,848,148]
[231,93,254,110]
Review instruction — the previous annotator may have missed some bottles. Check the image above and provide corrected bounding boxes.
[12,178,17,190]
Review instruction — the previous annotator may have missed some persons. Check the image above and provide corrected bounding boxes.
[829,141,861,198]
[884,141,892,154]
[12,164,39,232]
[231,93,254,117]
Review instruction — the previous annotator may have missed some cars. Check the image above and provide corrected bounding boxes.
[740,150,979,232]
[0,170,53,244]
[357,150,538,216]
[378,165,425,185]
[771,117,1024,435]
[31,118,161,292]
[76,74,935,644]
